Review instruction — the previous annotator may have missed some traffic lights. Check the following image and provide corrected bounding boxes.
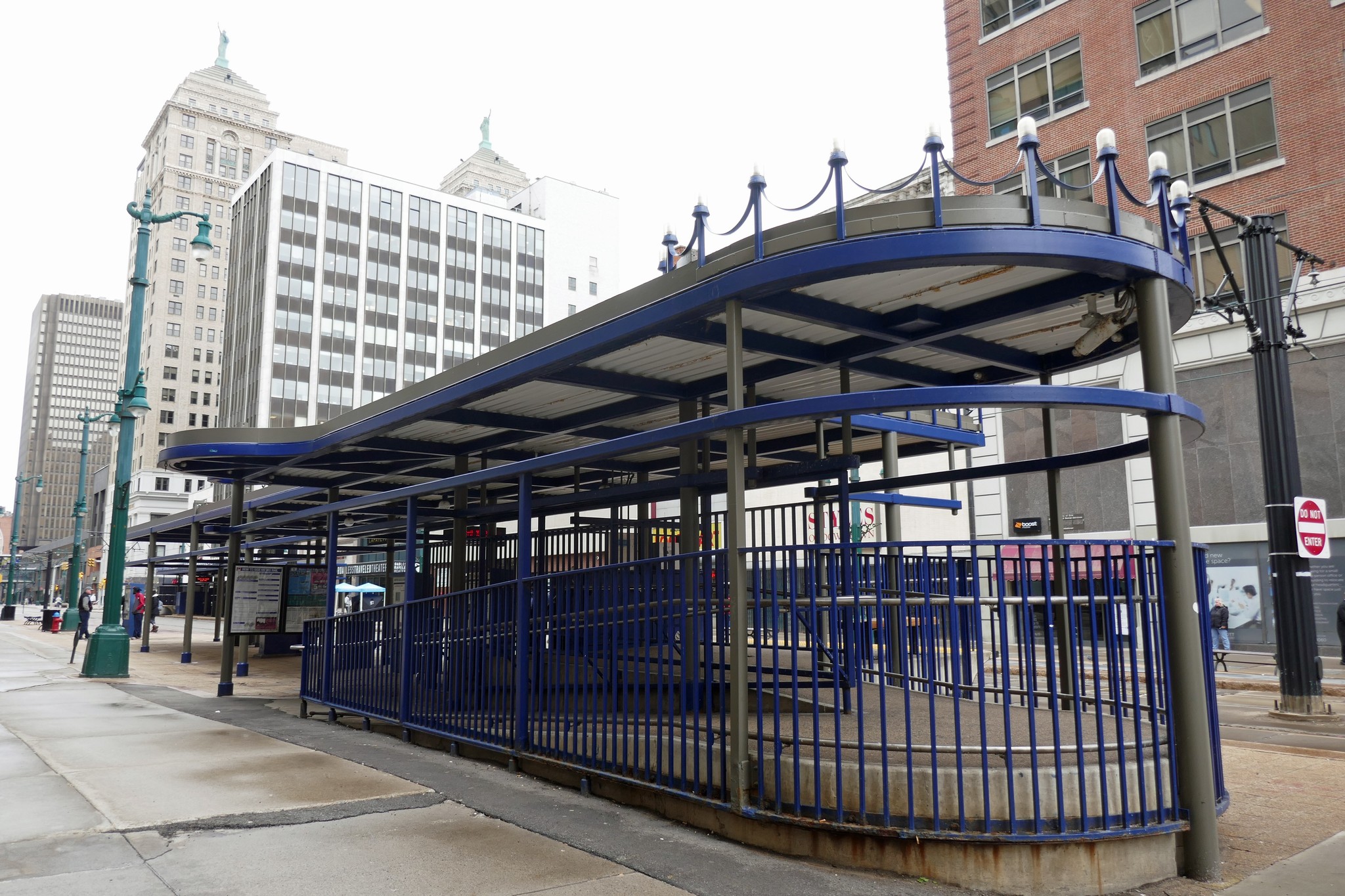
[98,583,102,589]
[62,562,69,570]
[91,558,96,567]
[79,572,84,581]
[55,585,59,591]
[88,558,92,567]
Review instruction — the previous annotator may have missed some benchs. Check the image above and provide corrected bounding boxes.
[24,616,43,630]
[1213,650,1279,676]
[837,616,940,659]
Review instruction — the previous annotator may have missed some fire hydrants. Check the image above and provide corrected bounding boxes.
[50,612,64,634]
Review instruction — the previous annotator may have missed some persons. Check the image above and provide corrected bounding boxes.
[1210,598,1230,655]
[2,598,4,604]
[122,595,125,626]
[344,595,352,613]
[131,586,145,640]
[79,586,97,640]
[150,589,159,632]
[24,597,34,605]
[1336,592,1345,665]
[1208,577,1262,620]
[53,596,62,608]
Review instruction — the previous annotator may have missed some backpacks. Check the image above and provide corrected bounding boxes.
[158,600,163,611]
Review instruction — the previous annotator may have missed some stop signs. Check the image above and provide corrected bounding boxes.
[1293,495,1331,559]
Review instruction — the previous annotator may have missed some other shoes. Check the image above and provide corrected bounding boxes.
[151,629,158,633]
[1339,660,1345,665]
[85,635,90,638]
[153,625,159,631]
[79,637,84,640]
[131,637,140,640]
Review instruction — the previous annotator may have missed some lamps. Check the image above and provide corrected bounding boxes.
[599,478,610,489]
[439,494,450,509]
[344,513,354,527]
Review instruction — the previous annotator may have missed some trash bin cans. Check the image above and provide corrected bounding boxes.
[42,609,61,632]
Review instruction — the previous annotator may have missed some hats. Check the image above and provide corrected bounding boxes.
[133,586,142,593]
[85,586,94,591]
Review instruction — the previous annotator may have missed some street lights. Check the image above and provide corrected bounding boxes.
[58,405,121,631]
[5,470,44,606]
[80,185,216,677]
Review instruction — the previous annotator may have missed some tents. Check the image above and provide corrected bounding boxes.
[334,582,386,618]
